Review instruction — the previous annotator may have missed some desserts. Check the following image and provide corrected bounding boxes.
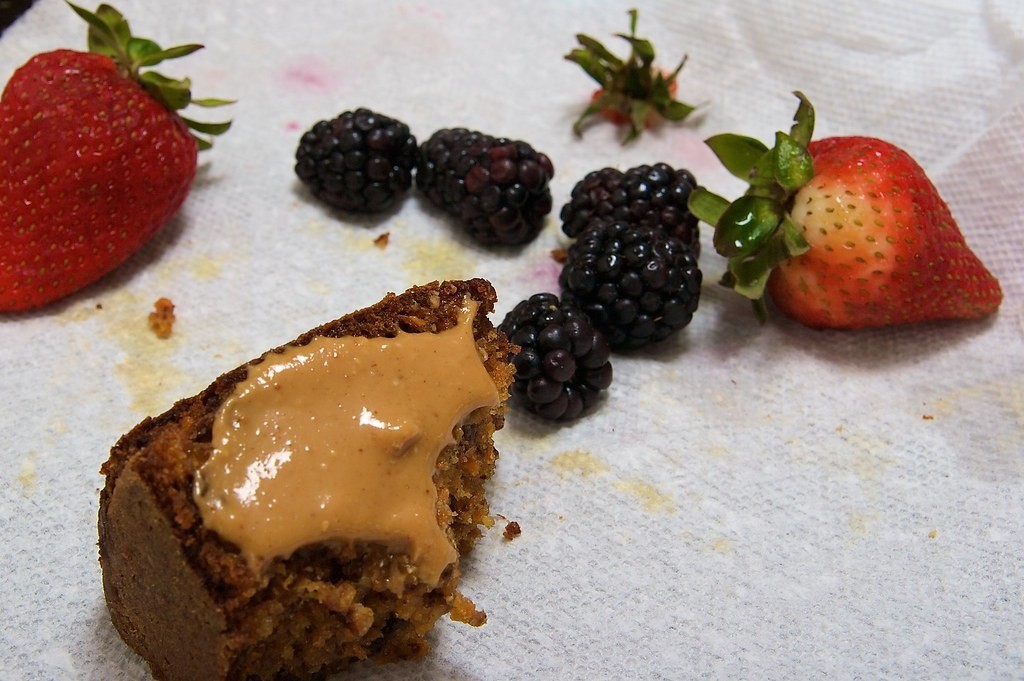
[96,278,523,681]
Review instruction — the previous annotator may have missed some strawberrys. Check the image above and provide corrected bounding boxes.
[686,90,1004,330]
[0,0,233,319]
